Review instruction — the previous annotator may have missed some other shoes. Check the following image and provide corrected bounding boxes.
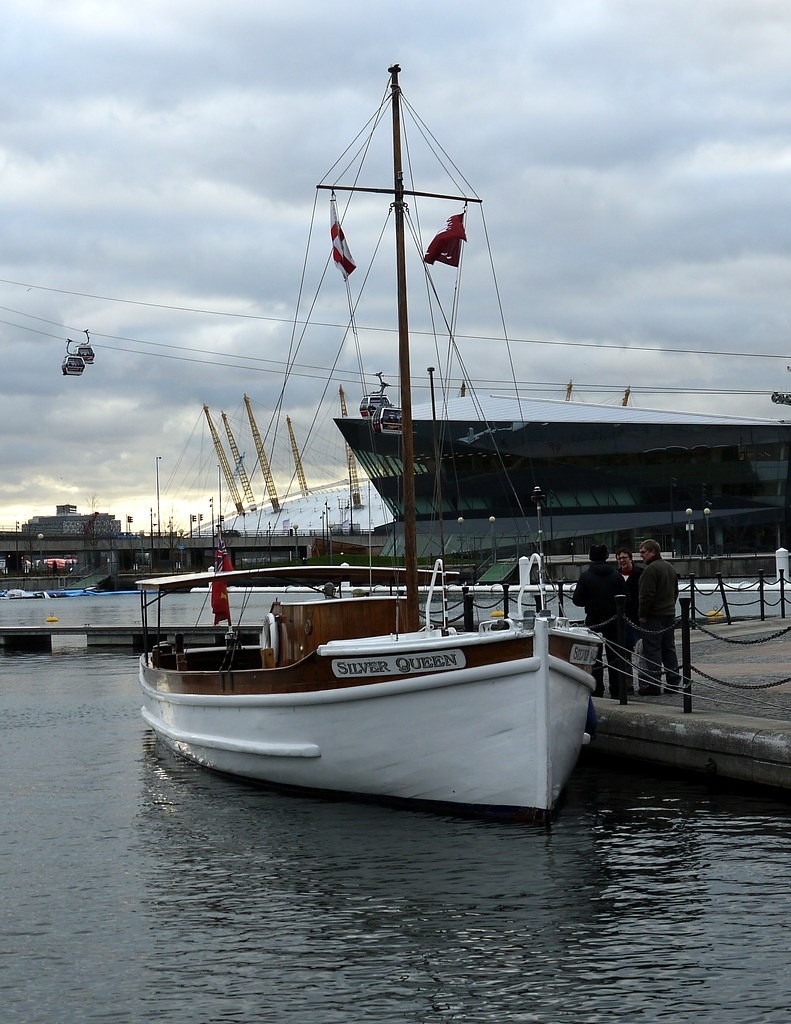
[638,686,661,696]
[592,690,603,697]
[625,687,635,695]
[611,693,620,699]
[663,684,678,694]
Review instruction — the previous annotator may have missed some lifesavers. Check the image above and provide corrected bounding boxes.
[260,611,280,667]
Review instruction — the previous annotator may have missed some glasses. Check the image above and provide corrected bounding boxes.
[617,556,630,560]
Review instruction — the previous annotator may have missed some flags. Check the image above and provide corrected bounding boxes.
[330,196,357,280]
[424,212,468,267]
[211,527,234,626]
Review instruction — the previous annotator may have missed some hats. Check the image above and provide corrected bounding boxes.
[590,543,609,561]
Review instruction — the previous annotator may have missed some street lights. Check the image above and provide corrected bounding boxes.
[704,507,711,559]
[292,523,299,565]
[37,533,44,592]
[686,508,694,560]
[138,530,145,581]
[488,516,496,564]
[177,529,186,574]
[326,521,335,565]
[457,517,464,581]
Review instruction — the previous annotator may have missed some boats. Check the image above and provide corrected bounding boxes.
[135,63,603,827]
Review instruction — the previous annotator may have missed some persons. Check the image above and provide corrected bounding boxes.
[637,538,681,695]
[52,560,60,577]
[572,542,647,701]
